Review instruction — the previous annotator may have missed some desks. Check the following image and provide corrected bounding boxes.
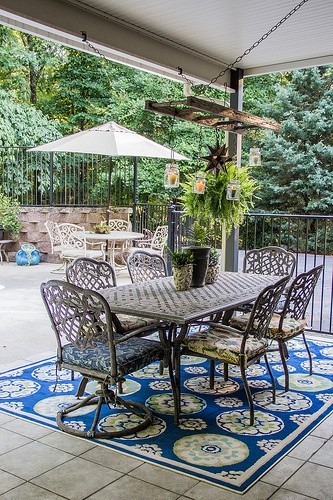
[72,230,144,279]
[54,277,299,427]
[0,240,12,264]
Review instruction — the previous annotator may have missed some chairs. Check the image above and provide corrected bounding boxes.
[174,274,292,425]
[101,219,132,270]
[128,225,168,272]
[40,280,162,438]
[224,263,325,392]
[127,249,168,283]
[242,246,296,366]
[44,220,66,274]
[66,257,164,376]
[55,223,106,271]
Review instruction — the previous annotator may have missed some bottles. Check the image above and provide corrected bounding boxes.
[226,180,240,200]
[249,148,261,166]
[192,173,206,194]
[164,163,179,188]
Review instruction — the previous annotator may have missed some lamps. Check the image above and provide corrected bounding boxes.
[144,85,281,202]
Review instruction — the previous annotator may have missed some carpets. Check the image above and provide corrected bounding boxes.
[0,324,333,494]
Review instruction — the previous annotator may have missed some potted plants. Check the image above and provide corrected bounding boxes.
[169,246,198,291]
[206,222,233,282]
[183,220,213,286]
[93,221,111,235]
